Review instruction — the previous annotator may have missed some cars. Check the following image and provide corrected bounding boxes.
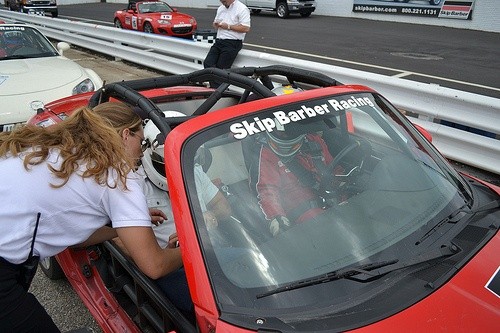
[5,0,58,18]
[238,0,317,18]
[114,1,198,38]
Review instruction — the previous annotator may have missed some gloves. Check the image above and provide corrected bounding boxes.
[270,216,289,236]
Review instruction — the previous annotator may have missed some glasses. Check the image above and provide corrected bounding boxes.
[131,131,149,152]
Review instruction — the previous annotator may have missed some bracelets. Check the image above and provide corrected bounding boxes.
[228,24,231,30]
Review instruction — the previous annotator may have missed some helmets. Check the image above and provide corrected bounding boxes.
[266,87,308,158]
[142,111,205,191]
[3,29,24,51]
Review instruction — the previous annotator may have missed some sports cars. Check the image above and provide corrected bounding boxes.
[1,21,104,138]
[21,64,500,333]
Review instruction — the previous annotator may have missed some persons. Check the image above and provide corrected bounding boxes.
[110,110,253,314]
[2,29,23,56]
[0,101,216,333]
[256,84,348,237]
[203,0,251,89]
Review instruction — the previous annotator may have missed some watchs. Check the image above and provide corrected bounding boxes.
[202,211,218,230]
